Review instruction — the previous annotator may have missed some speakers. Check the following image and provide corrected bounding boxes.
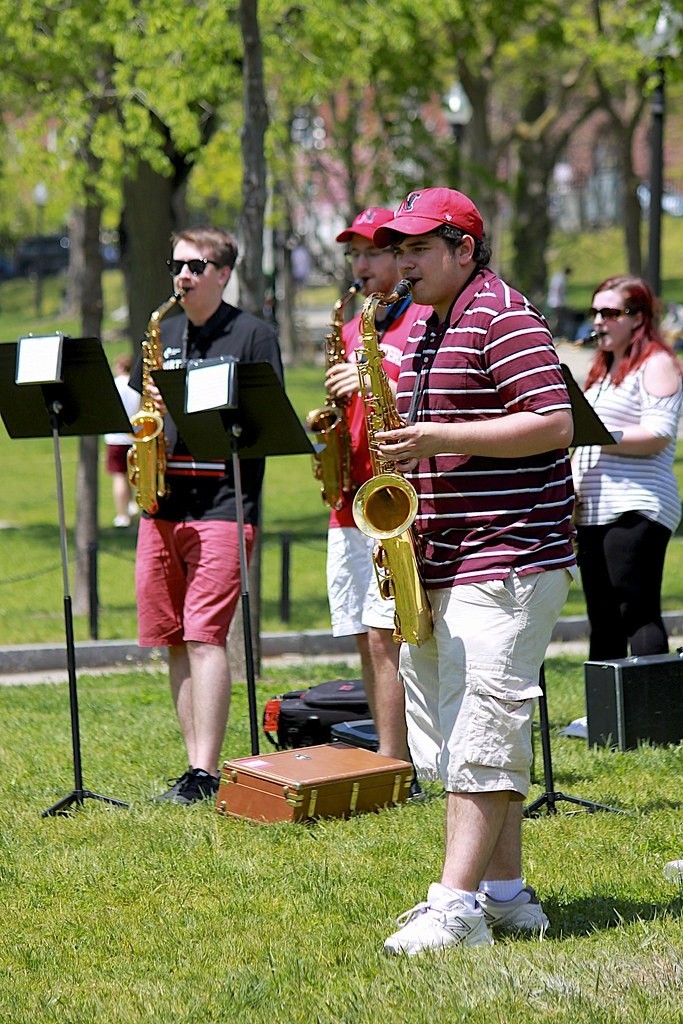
[584,652,683,752]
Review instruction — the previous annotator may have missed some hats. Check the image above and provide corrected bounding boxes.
[335,208,395,244]
[375,187,483,250]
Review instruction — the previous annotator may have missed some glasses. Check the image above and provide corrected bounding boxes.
[343,249,393,263]
[589,307,636,321]
[165,258,221,277]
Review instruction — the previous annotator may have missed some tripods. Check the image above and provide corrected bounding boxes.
[524,661,641,815]
[0,335,132,819]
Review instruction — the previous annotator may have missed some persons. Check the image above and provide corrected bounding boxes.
[104,356,141,528]
[128,227,286,806]
[373,188,575,954]
[320,206,435,802]
[550,266,571,340]
[564,276,682,663]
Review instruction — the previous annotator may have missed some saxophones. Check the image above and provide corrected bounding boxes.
[352,276,437,650]
[126,286,194,516]
[304,276,368,513]
[553,331,610,560]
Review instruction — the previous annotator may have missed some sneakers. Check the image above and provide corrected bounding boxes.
[150,772,191,804]
[481,886,550,933]
[567,717,587,742]
[384,880,493,955]
[171,768,222,807]
